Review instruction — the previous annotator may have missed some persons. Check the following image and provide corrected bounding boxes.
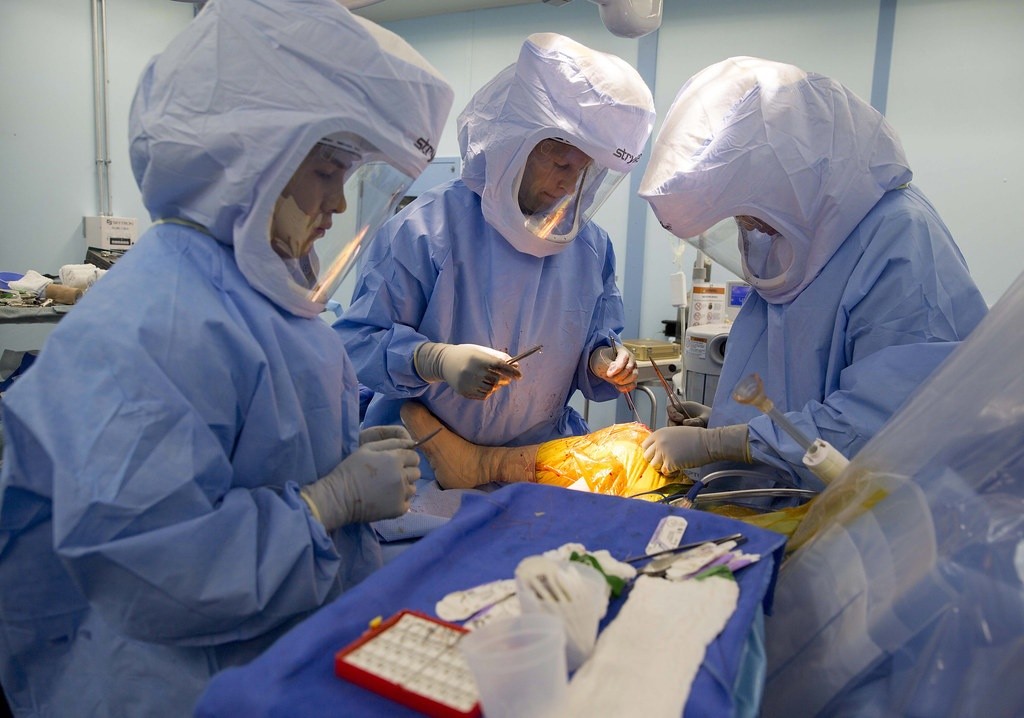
[396,398,1024,716]
[0,0,418,718]
[327,30,637,486]
[637,56,1003,522]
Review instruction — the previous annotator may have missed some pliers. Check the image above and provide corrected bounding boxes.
[608,335,636,410]
[506,345,543,368]
[647,354,691,420]
[617,531,743,571]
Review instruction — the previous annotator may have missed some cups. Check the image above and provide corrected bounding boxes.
[517,560,609,670]
[461,615,570,717]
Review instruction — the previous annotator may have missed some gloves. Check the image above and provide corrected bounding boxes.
[412,338,523,401]
[298,436,419,529]
[590,342,638,392]
[664,400,712,427]
[360,424,410,454]
[641,421,751,475]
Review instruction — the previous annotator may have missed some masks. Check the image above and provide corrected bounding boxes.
[740,229,780,277]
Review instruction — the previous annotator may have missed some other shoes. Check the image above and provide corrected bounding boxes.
[399,401,541,488]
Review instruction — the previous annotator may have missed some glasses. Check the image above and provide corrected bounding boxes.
[732,215,762,231]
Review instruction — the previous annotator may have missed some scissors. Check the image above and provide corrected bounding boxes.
[407,427,442,450]
